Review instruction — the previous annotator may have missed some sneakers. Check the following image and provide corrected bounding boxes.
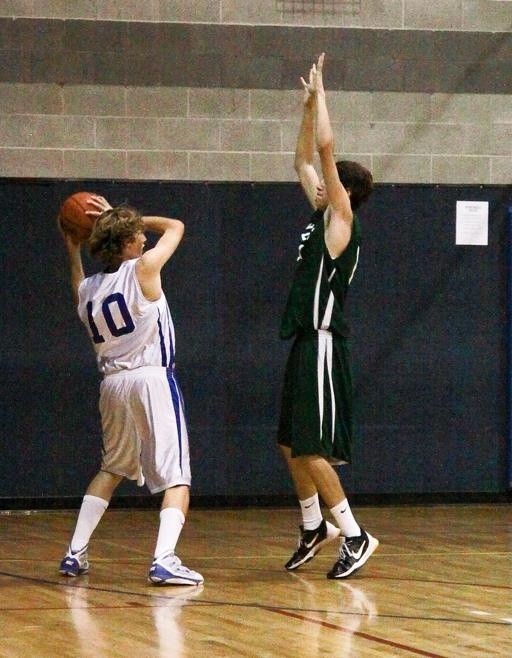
[147,555,205,586]
[326,525,380,580]
[284,517,341,571]
[58,541,90,577]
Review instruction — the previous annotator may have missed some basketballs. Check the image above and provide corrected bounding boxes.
[60,191,106,239]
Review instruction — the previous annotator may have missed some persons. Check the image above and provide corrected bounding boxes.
[275,51,383,580]
[55,193,206,588]
[285,566,376,657]
[55,565,205,657]
